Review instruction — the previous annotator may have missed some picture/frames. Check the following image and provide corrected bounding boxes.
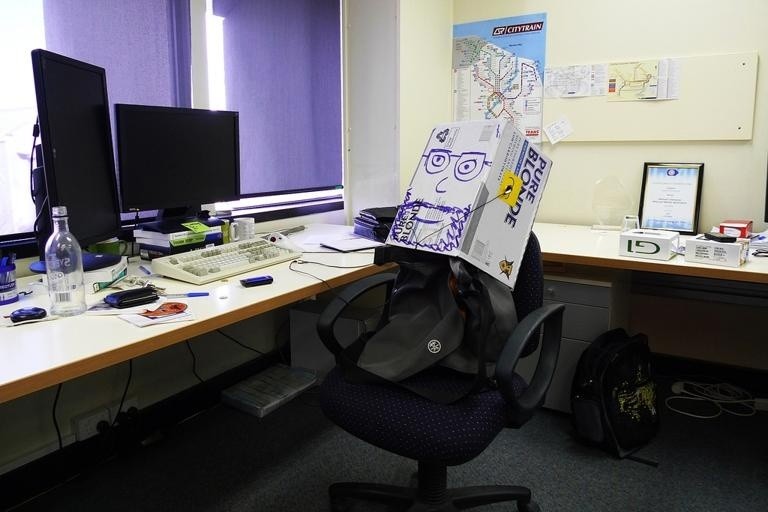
[636,163,704,234]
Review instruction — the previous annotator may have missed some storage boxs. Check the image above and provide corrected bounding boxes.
[380,116,554,292]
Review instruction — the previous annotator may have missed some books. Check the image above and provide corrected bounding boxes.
[132,218,221,241]
[136,231,224,248]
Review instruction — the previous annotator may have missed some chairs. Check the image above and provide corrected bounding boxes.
[311,220,566,512]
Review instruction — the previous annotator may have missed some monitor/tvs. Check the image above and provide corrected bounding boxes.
[29,48,123,274]
[113,103,241,234]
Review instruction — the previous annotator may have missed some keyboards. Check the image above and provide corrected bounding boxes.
[151,237,303,286]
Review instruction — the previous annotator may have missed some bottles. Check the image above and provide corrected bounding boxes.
[45,206,87,317]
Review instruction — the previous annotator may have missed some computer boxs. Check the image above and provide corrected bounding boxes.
[289,303,377,387]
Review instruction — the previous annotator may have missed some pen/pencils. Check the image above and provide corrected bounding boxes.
[140,266,151,275]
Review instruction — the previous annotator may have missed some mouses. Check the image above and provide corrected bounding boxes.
[267,232,304,253]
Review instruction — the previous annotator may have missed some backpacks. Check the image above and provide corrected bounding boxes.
[569,326,663,469]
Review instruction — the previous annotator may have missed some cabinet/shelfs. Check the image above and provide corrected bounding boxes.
[540,265,632,421]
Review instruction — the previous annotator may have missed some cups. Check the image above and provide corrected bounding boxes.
[0,263,19,306]
[229,217,256,243]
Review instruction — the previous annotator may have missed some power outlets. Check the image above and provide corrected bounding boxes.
[74,396,143,440]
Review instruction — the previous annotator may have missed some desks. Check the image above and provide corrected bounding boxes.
[1,213,768,511]
